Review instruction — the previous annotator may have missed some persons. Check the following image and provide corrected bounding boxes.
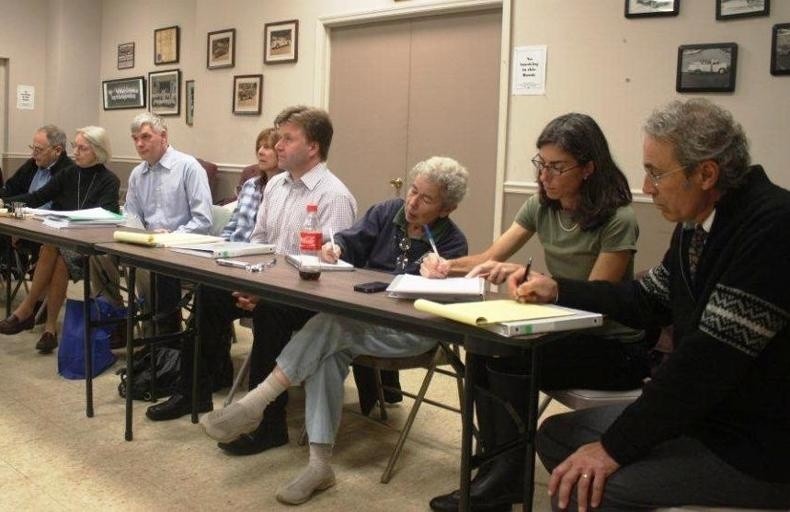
[198,155,470,507]
[207,125,287,392]
[141,102,358,457]
[506,93,790,512]
[90,109,215,370]
[0,126,122,353]
[418,111,646,512]
[0,123,72,277]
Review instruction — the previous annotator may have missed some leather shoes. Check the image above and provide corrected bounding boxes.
[427,482,512,512]
[36,331,59,350]
[0,313,36,334]
[217,424,291,455]
[451,460,536,503]
[146,394,216,418]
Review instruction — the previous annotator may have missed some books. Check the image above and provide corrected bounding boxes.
[38,205,124,231]
[285,253,355,273]
[413,292,576,327]
[169,240,277,259]
[386,271,487,302]
[112,229,225,249]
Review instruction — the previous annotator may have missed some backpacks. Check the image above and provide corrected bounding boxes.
[115,340,182,401]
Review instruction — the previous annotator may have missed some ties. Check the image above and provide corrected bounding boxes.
[687,224,707,277]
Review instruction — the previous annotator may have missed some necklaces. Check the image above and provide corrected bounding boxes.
[556,208,581,234]
[73,171,98,211]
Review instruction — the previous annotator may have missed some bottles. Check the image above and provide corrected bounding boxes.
[297,204,324,282]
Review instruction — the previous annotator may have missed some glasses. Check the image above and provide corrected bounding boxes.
[532,154,585,177]
[643,164,692,190]
[397,237,411,273]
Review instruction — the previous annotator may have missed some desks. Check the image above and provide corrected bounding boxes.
[94,236,607,512]
[0,209,162,416]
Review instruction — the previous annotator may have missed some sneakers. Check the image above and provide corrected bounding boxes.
[200,399,265,445]
[274,463,336,504]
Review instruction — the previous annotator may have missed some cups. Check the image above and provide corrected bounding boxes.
[10,201,26,220]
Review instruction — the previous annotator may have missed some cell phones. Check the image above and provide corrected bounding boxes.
[354,282,389,293]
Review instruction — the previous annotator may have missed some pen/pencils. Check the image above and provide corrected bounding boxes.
[521,257,533,284]
[423,223,444,262]
[328,228,336,250]
[217,260,250,270]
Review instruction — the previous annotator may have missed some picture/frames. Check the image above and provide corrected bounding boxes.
[770,23,790,75]
[102,69,195,125]
[233,75,261,115]
[676,43,737,92]
[624,0,679,19]
[207,29,235,70]
[716,0,770,20]
[118,42,134,70]
[264,19,299,65]
[153,26,179,65]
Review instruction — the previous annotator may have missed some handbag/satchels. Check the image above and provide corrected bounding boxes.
[54,295,115,380]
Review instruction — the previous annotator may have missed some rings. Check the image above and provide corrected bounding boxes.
[580,473,593,482]
